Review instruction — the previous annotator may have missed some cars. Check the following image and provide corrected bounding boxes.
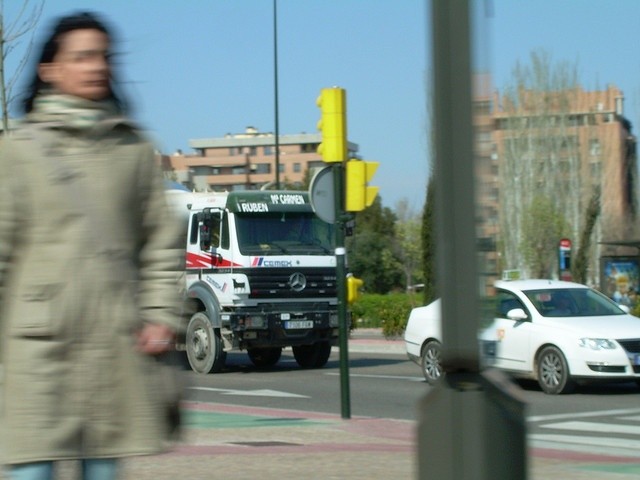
[405,269,640,395]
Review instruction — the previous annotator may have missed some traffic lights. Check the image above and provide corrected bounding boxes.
[316,86,347,162]
[345,158,381,211]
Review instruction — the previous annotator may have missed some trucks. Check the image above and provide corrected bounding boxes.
[165,189,351,374]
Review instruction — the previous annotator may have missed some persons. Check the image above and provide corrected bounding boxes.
[0,15,188,479]
[552,294,579,314]
[276,216,310,241]
